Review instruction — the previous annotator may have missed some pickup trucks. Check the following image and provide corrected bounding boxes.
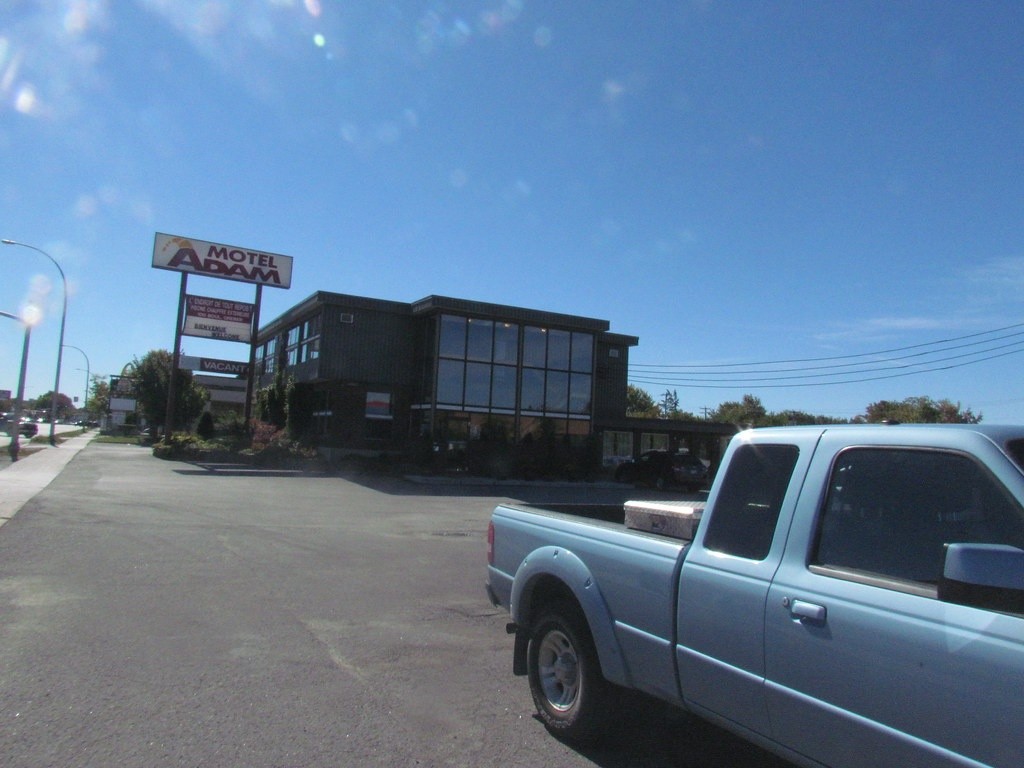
[487,424,1023,766]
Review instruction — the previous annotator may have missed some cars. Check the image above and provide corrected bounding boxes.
[633,446,714,493]
[0,412,39,439]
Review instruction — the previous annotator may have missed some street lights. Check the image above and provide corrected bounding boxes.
[61,344,89,433]
[1,238,69,445]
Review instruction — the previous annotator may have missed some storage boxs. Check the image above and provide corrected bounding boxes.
[624,499,708,540]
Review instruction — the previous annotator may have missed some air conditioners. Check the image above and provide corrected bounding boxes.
[339,312,354,324]
